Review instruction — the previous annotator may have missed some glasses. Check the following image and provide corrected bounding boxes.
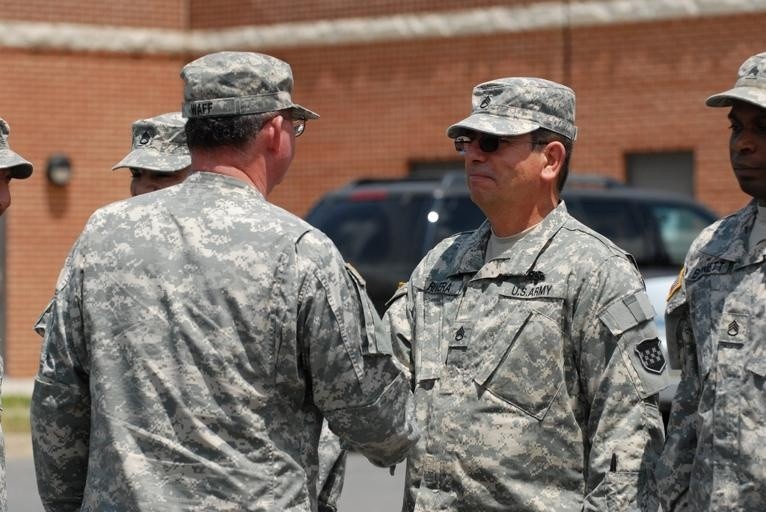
[454,135,549,152]
[281,113,306,138]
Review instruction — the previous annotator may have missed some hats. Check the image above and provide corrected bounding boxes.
[1,118,34,180]
[178,49,322,124]
[446,76,578,140]
[703,51,766,111]
[110,109,192,173]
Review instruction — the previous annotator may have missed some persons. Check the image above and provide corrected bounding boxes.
[382,77,670,512]
[0,118,32,512]
[658,51,766,512]
[112,112,347,512]
[31,51,421,512]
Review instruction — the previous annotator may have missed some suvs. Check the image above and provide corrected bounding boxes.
[299,160,731,355]
